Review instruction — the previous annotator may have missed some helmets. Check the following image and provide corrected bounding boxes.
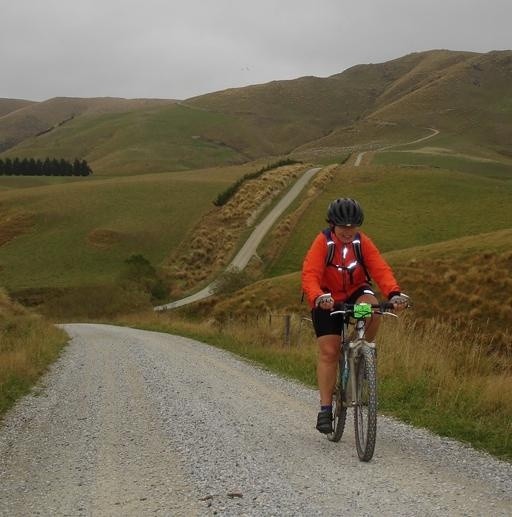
[327,198,365,228]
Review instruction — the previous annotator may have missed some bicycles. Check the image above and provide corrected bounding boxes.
[327,300,416,462]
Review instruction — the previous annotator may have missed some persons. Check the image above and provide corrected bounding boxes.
[301,198,409,435]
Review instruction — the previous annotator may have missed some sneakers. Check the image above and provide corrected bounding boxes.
[316,411,332,433]
